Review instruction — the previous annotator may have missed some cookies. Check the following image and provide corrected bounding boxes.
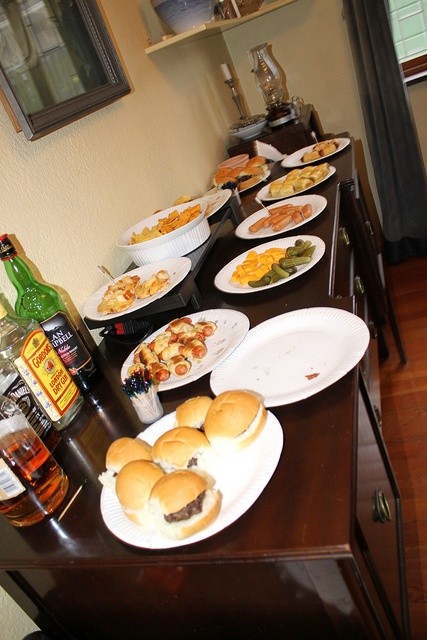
[128,203,201,244]
[174,196,193,203]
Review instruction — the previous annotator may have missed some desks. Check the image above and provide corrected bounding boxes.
[226,102,325,164]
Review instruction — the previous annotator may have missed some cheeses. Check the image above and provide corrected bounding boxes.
[232,248,287,285]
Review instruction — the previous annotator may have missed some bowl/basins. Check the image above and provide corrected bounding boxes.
[228,117,268,140]
[217,154,249,170]
[115,198,211,267]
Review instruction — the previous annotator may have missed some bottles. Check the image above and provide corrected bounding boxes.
[0,233,103,395]
[0,354,52,441]
[0,294,85,433]
[0,394,70,530]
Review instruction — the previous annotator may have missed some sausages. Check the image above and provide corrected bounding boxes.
[248,204,312,233]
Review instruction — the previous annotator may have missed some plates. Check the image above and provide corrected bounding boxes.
[256,165,337,201]
[237,169,271,193]
[234,194,328,239]
[214,235,326,295]
[193,189,233,218]
[280,138,351,168]
[81,257,192,322]
[100,409,284,550]
[209,307,371,408]
[120,309,250,393]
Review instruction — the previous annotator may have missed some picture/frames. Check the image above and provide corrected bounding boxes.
[0,0,132,142]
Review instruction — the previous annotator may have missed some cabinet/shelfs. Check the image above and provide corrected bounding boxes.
[141,0,315,57]
[0,131,412,640]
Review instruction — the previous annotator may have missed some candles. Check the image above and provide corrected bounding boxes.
[218,61,231,83]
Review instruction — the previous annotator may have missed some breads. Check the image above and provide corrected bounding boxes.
[127,318,216,384]
[300,142,336,162]
[268,163,329,197]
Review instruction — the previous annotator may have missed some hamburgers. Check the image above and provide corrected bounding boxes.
[115,460,164,526]
[147,470,219,540]
[215,156,268,197]
[151,427,216,488]
[203,390,266,450]
[174,396,212,431]
[98,437,152,494]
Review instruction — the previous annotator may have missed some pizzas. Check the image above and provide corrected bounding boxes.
[136,272,170,299]
[96,275,140,314]
